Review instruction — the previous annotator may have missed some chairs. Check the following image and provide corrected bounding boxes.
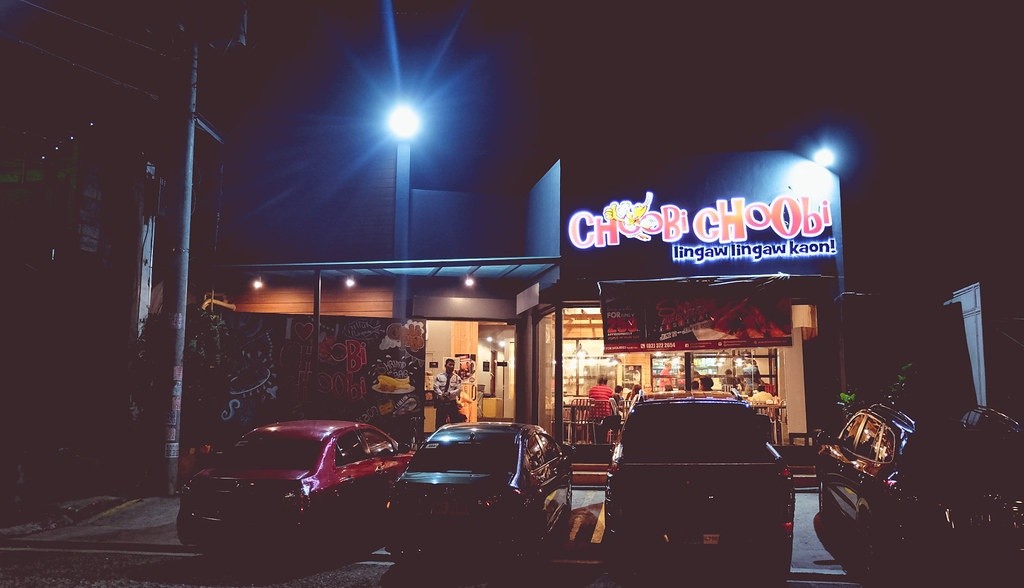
[557,397,631,445]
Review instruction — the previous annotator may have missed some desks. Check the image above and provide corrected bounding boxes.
[750,402,785,443]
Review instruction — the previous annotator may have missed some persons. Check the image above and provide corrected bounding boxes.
[659,359,761,393]
[613,385,642,401]
[589,374,622,444]
[433,359,462,430]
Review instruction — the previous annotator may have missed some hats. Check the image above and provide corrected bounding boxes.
[700,377,713,387]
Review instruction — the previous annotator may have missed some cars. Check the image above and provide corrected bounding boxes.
[175,419,417,546]
[384,422,574,542]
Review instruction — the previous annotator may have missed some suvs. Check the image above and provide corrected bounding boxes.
[599,390,796,562]
[811,401,1024,577]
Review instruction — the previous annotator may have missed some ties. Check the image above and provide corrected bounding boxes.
[444,376,451,392]
